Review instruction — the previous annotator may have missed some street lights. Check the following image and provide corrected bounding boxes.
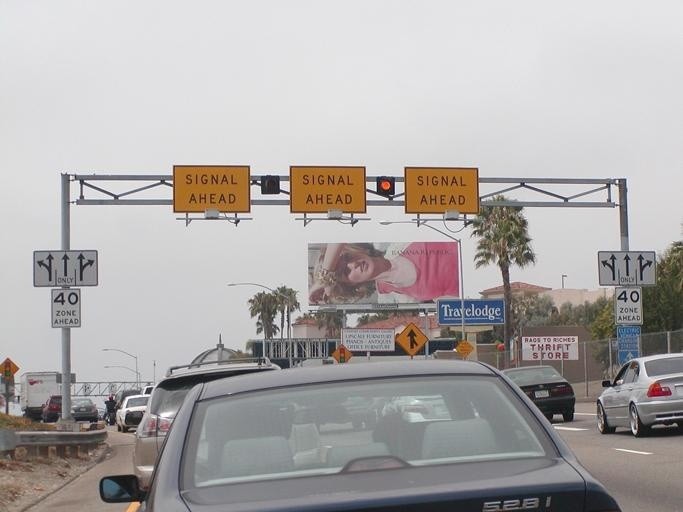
[100,348,155,384]
[561,275,567,289]
[378,221,466,360]
[227,282,292,368]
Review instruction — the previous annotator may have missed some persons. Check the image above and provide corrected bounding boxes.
[102,394,116,422]
[307,243,459,305]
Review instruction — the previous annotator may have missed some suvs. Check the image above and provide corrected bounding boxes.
[42,394,62,422]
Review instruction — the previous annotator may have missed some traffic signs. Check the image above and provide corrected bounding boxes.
[52,290,80,326]
[596,249,656,286]
[615,286,642,325]
[618,327,641,365]
[33,250,98,286]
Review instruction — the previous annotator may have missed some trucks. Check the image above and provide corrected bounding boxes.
[20,371,61,418]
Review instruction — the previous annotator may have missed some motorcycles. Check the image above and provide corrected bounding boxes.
[105,413,115,425]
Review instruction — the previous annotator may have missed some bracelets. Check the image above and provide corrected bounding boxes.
[316,268,337,286]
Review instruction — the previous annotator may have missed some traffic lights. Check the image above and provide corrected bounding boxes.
[261,176,279,194]
[376,177,395,195]
[338,346,345,363]
[4,360,10,378]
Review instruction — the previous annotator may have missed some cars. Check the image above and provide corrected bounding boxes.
[596,351,683,437]
[71,398,98,423]
[130,363,286,494]
[97,362,621,510]
[502,364,577,423]
[109,363,451,437]
